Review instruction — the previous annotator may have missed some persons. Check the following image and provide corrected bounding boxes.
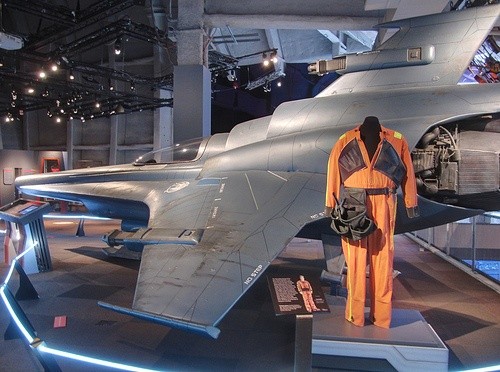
[296,275,321,313]
[324,116,420,329]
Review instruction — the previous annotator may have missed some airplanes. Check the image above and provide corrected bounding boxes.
[14,3,500,340]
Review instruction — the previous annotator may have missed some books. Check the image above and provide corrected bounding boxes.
[267,270,330,316]
